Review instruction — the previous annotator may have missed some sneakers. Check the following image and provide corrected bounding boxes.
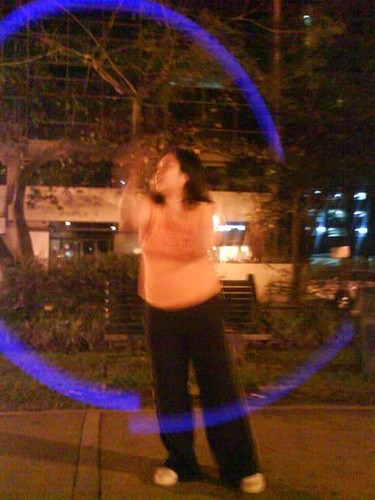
[240,472,265,494]
[153,467,178,486]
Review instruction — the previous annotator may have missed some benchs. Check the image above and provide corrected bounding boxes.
[100,272,275,378]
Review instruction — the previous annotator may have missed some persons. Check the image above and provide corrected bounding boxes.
[119,142,265,494]
